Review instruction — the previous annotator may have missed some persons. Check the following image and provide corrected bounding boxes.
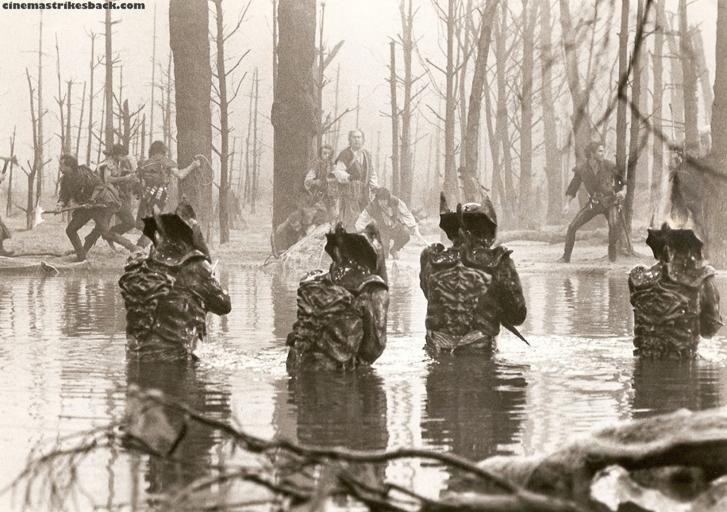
[299,144,336,237]
[555,141,627,264]
[417,192,527,355]
[52,154,136,263]
[134,142,202,256]
[356,186,420,260]
[282,219,387,373]
[0,171,15,259]
[115,197,231,363]
[84,144,137,252]
[629,218,723,364]
[334,127,377,227]
